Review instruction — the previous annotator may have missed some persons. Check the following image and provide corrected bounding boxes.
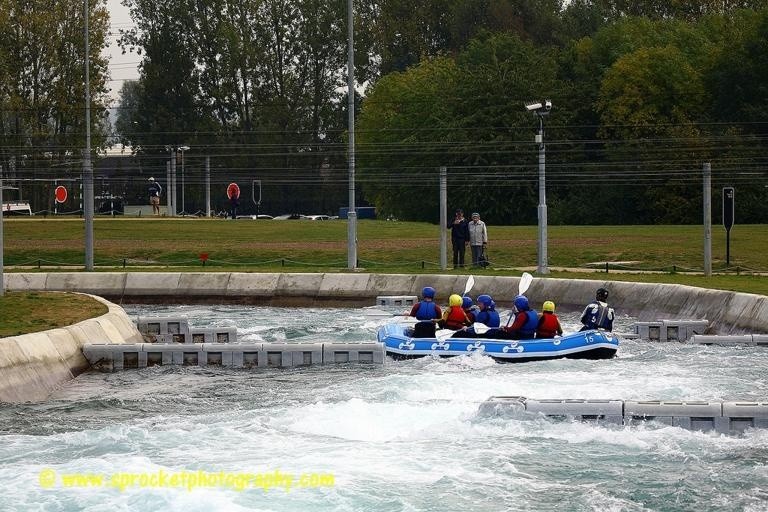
[536,300,563,338]
[403,286,444,337]
[450,294,501,338]
[447,208,471,269]
[485,295,540,339]
[147,176,163,216]
[461,296,480,324]
[577,287,616,332]
[438,294,472,330]
[230,189,238,219]
[468,212,488,265]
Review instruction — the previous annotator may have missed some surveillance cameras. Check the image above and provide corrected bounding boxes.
[525,103,542,111]
[545,101,552,110]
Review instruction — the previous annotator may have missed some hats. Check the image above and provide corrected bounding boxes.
[472,213,478,218]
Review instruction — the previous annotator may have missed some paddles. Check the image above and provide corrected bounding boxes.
[506,272,534,327]
[475,322,502,333]
[435,325,474,342]
[398,316,431,327]
[462,275,475,297]
[366,310,411,320]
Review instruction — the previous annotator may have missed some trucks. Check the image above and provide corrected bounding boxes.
[339,205,379,220]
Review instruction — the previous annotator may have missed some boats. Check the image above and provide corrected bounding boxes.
[375,317,618,363]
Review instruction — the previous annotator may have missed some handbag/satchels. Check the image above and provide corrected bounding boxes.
[479,256,489,267]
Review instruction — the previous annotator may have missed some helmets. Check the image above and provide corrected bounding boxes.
[422,287,435,298]
[542,301,555,312]
[449,294,495,308]
[513,295,529,311]
[597,288,608,299]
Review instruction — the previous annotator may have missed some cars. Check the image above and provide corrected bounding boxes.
[225,212,338,222]
